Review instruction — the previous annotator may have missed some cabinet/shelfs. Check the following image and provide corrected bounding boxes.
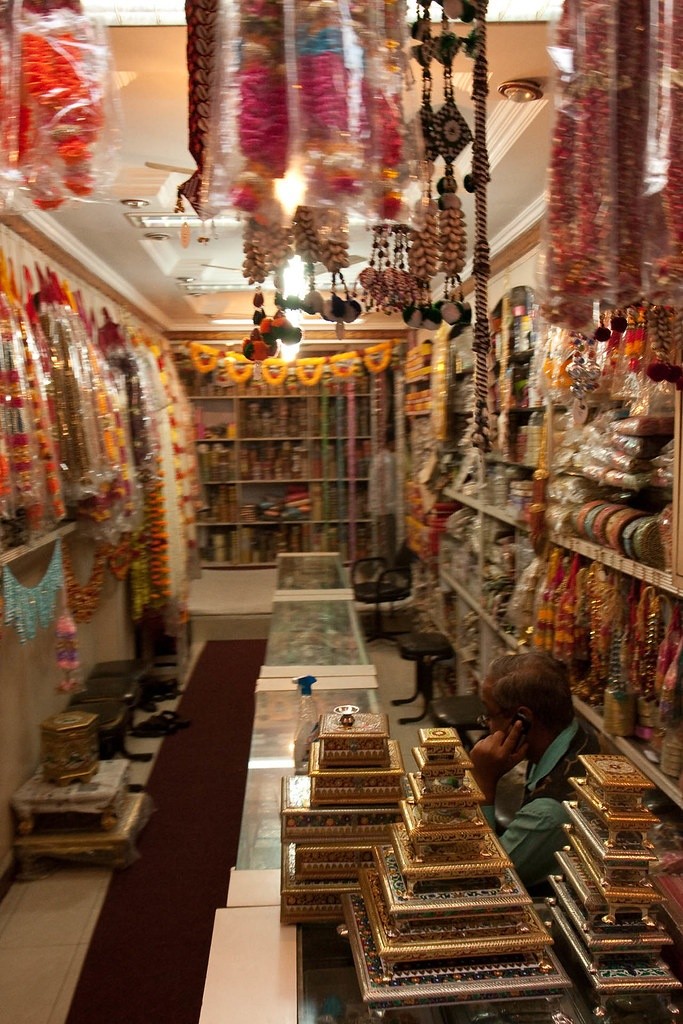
[403,287,683,975]
[197,552,381,1024]
[186,392,378,569]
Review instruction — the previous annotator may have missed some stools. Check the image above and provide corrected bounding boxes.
[392,633,454,729]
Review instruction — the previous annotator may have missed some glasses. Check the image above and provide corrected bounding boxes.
[479,712,500,727]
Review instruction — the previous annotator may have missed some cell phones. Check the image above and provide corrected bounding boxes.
[504,713,530,752]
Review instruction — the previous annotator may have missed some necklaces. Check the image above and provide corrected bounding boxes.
[0,0,683,747]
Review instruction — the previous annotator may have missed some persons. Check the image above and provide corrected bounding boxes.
[469,649,604,891]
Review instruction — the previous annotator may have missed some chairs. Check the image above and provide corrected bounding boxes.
[350,545,413,644]
